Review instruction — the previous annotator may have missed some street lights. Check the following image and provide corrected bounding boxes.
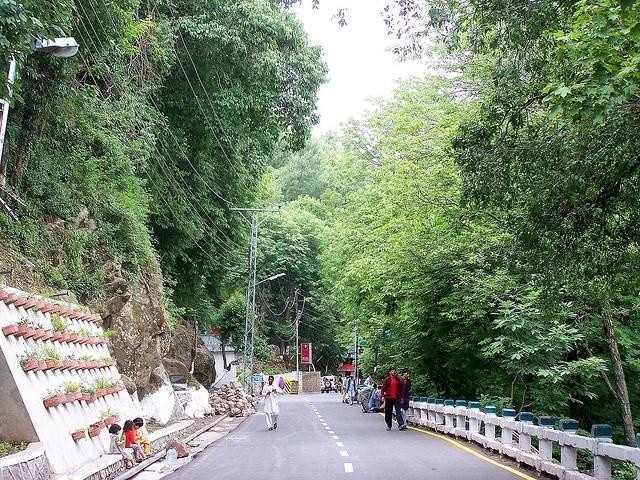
[296,309,302,389]
[237,272,286,393]
[354,325,358,379]
[1,32,83,161]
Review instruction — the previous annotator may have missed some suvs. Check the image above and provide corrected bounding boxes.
[320,375,342,394]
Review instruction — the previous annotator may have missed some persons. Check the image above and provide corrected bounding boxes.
[262,376,283,431]
[123,420,147,461]
[109,425,138,469]
[278,376,285,390]
[132,418,154,457]
[335,368,411,431]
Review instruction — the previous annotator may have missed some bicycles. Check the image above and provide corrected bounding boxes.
[345,384,377,412]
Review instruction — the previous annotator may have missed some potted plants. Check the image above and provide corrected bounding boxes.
[1,284,125,440]
